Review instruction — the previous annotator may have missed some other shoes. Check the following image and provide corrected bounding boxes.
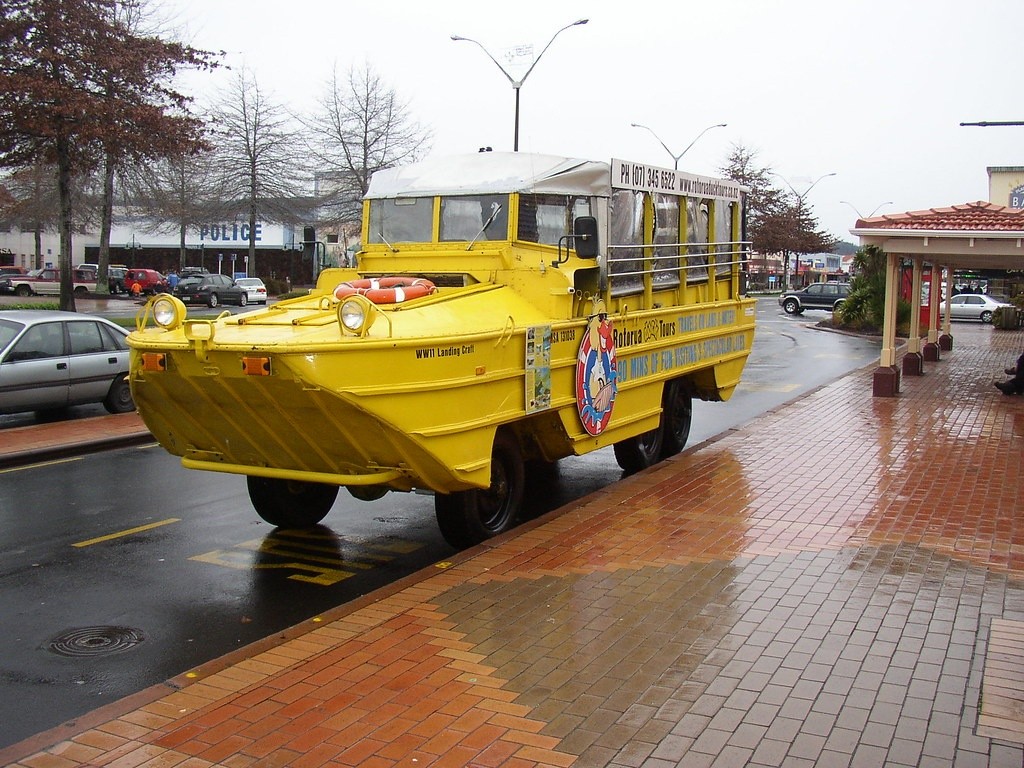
[1004,366,1017,374]
[994,379,1016,395]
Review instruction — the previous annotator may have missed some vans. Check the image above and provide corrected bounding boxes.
[124,268,169,296]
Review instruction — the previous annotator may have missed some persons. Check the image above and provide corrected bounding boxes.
[994,350,1024,395]
[951,284,983,295]
[130,280,143,298]
[152,271,179,296]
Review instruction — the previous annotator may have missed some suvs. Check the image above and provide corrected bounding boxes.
[180,266,211,276]
[78,264,99,277]
[109,268,132,295]
[4,267,99,298]
[774,280,854,317]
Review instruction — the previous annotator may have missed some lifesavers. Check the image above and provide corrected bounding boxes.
[333,276,435,304]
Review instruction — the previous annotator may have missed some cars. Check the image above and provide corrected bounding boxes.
[1,309,138,418]
[235,277,270,305]
[170,274,249,309]
[0,265,27,275]
[940,294,1012,324]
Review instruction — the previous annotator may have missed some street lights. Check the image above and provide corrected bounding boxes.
[282,233,304,293]
[630,122,728,170]
[767,170,838,285]
[124,233,142,269]
[449,19,590,152]
